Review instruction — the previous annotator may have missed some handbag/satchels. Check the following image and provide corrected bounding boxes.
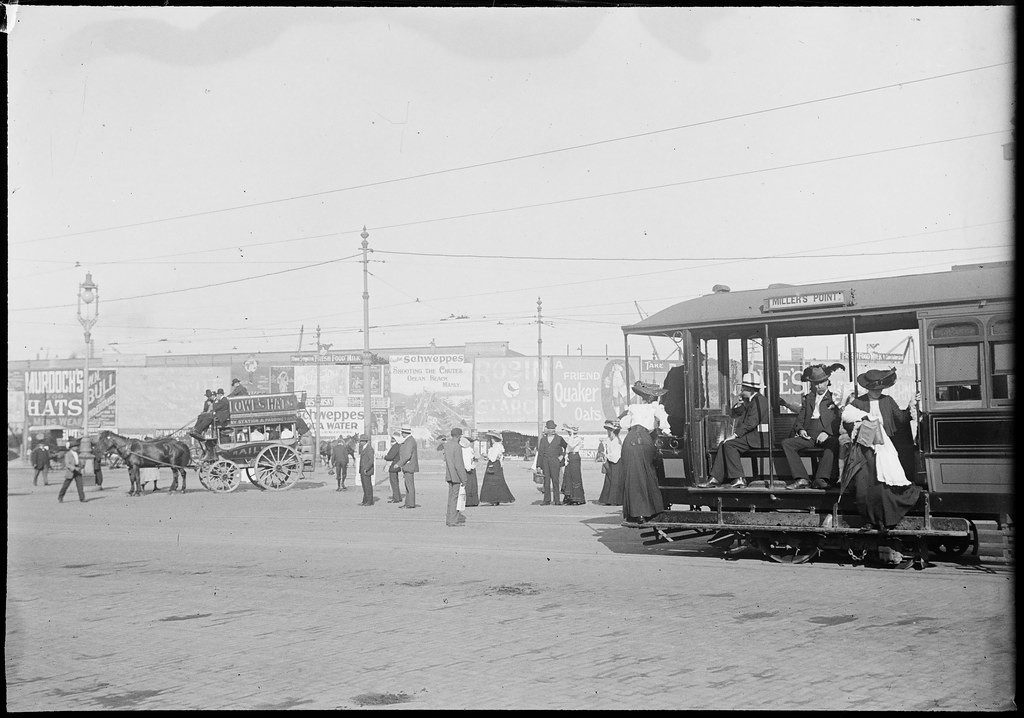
[533,472,545,484]
[488,460,495,474]
[856,419,885,447]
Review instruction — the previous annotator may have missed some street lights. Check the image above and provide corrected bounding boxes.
[77,270,99,475]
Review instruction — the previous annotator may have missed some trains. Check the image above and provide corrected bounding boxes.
[619,259,1015,572]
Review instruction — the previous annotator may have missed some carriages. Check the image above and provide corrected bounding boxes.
[93,389,318,495]
[28,425,98,471]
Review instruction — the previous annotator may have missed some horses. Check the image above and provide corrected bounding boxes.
[319,432,360,468]
[97,430,190,498]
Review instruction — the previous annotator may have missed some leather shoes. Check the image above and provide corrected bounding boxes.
[811,478,832,490]
[724,477,747,489]
[696,476,721,489]
[785,478,811,490]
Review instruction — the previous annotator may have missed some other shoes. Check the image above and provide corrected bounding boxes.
[877,521,889,533]
[861,521,873,531]
[337,479,647,530]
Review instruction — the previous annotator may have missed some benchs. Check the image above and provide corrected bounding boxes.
[211,415,230,432]
[704,410,914,490]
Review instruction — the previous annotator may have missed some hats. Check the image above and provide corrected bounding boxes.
[604,418,623,430]
[561,423,580,433]
[486,430,502,440]
[545,420,557,429]
[465,437,476,442]
[857,366,898,390]
[805,367,829,382]
[69,442,80,448]
[451,428,463,436]
[632,381,670,399]
[358,434,368,441]
[401,425,412,434]
[391,431,403,444]
[203,377,243,397]
[736,373,767,389]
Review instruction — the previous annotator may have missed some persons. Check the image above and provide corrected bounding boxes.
[598,420,623,506]
[595,439,605,462]
[394,425,419,509]
[90,445,103,491]
[560,423,585,505]
[779,363,845,414]
[621,380,672,529]
[444,428,565,526]
[331,439,349,491]
[698,372,775,488]
[537,420,567,505]
[358,434,374,506]
[605,362,635,418]
[781,367,841,490]
[31,439,51,485]
[838,365,922,532]
[192,378,313,442]
[383,431,402,503]
[139,437,161,491]
[57,440,88,503]
